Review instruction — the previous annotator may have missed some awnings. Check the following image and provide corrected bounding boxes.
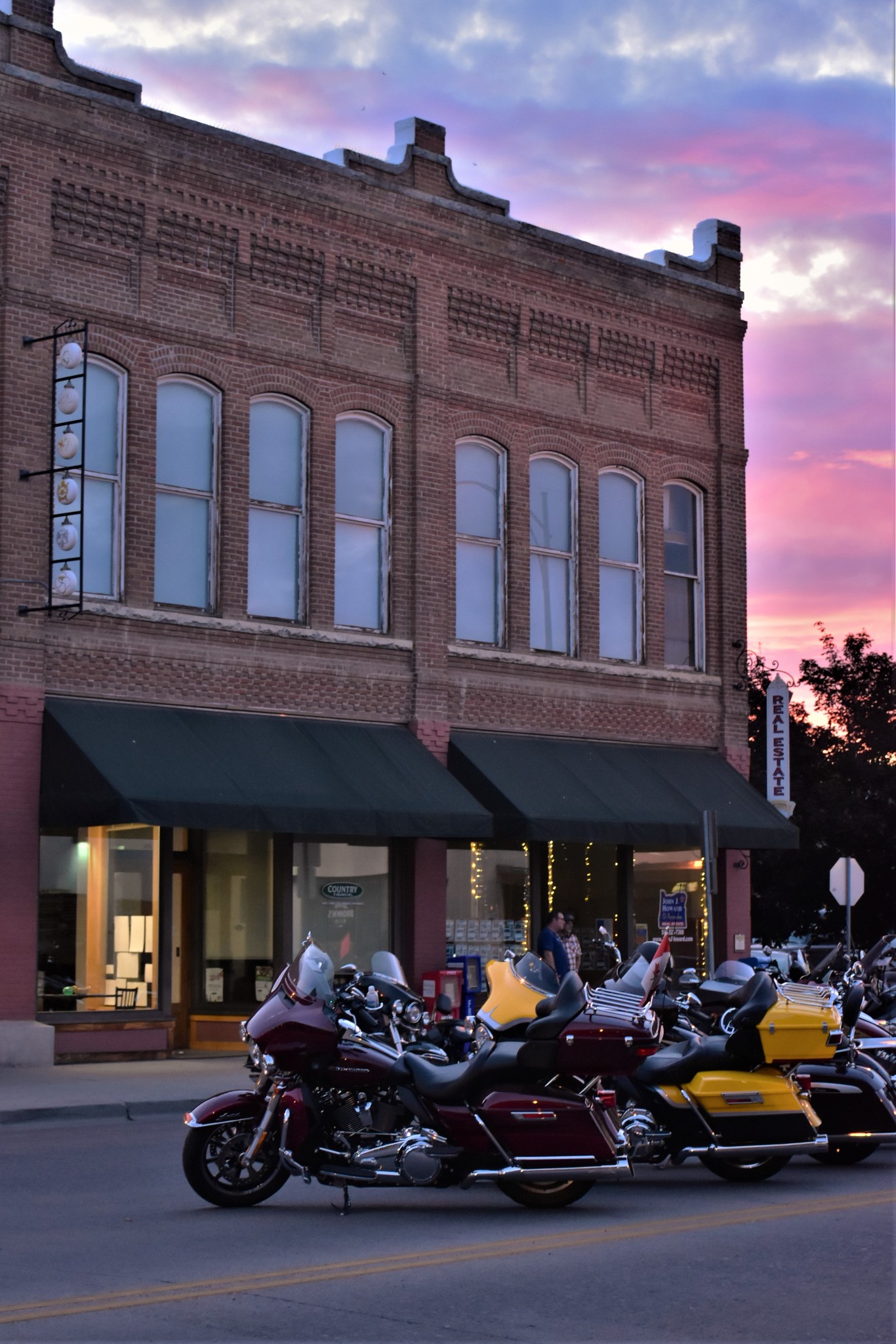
[37,694,496,840]
[447,727,799,849]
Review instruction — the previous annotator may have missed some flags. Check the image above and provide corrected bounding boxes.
[639,933,671,1007]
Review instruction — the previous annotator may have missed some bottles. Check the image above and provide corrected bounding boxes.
[62,986,74,995]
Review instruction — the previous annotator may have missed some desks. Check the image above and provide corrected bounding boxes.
[36,994,122,1011]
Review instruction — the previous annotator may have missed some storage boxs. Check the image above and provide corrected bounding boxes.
[758,989,843,1063]
[559,1003,664,1077]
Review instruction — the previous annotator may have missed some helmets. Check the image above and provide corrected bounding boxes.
[563,912,574,921]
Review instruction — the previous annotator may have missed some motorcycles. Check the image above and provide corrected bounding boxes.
[183,928,896,1209]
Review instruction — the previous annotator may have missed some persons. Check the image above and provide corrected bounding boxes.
[539,906,625,988]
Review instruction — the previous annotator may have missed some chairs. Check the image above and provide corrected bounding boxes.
[115,987,138,1009]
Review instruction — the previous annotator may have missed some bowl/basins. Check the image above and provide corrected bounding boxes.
[73,986,91,994]
